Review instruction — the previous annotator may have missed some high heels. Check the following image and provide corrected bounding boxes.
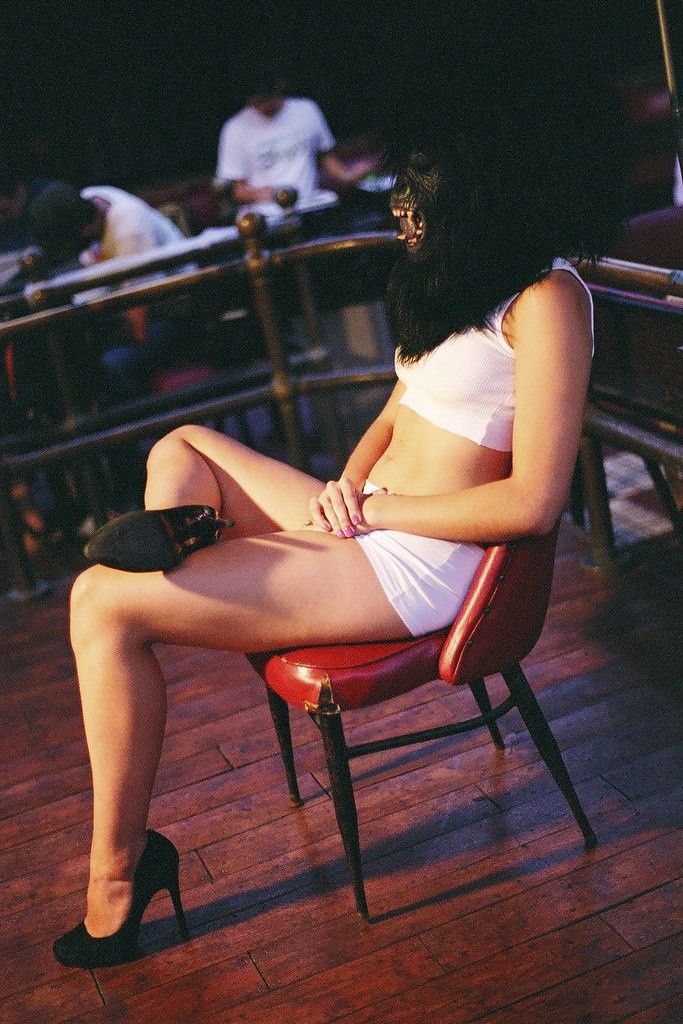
[83,504,234,573]
[54,828,185,969]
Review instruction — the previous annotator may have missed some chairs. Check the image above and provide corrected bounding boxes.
[244,463,597,922]
[0,87,683,569]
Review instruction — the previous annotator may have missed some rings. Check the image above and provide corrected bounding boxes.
[305,520,313,526]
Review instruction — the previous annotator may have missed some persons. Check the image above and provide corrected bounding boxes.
[0,170,225,310]
[211,70,384,232]
[52,89,595,969]
